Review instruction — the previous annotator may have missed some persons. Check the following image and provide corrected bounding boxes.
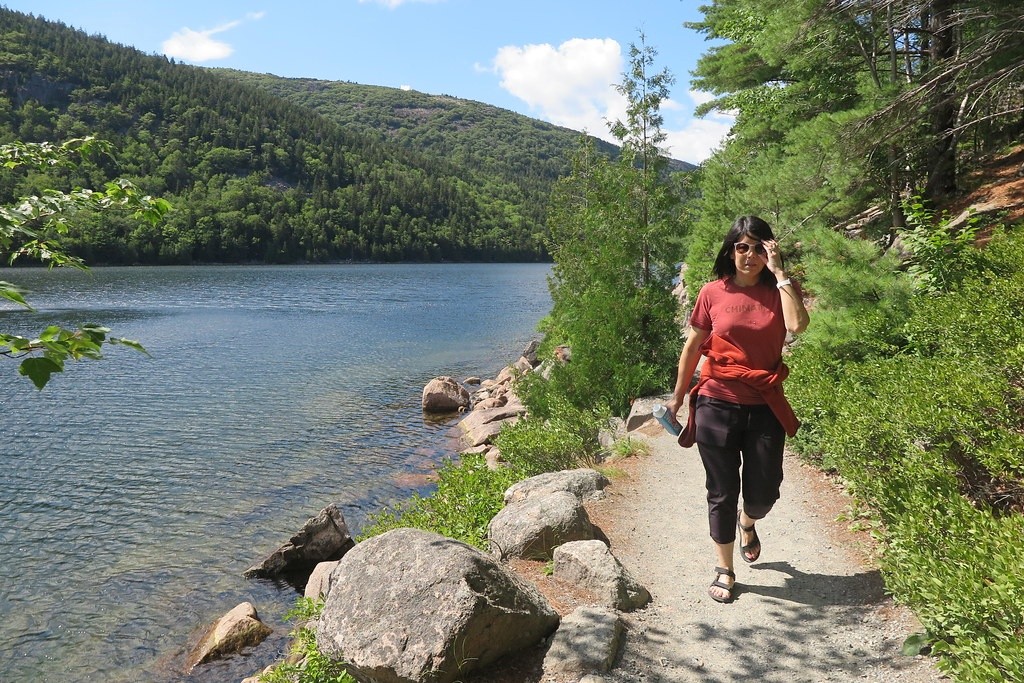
[660,215,810,603]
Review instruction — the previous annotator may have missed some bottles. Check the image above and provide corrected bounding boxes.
[653,404,683,437]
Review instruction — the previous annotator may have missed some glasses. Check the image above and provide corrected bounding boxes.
[733,243,764,255]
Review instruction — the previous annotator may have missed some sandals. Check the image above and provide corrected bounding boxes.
[708,566,736,602]
[737,509,761,563]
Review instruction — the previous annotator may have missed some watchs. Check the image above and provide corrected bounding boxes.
[776,278,793,289]
